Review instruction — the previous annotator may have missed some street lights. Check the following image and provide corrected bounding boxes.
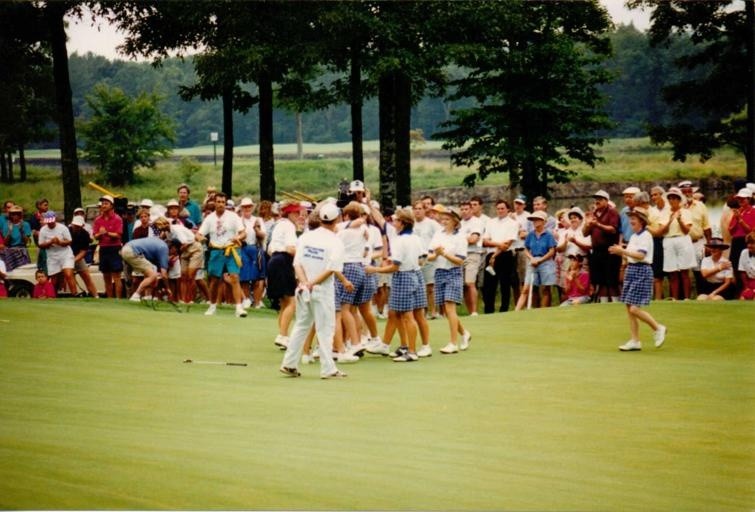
[211,132,220,169]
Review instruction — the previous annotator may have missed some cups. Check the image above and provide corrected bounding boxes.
[485,266,497,276]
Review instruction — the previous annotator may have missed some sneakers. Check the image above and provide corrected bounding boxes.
[654,324,667,348]
[274,335,290,349]
[320,369,347,380]
[619,341,642,352]
[129,287,267,309]
[337,352,359,363]
[312,333,381,360]
[366,343,390,357]
[204,307,217,316]
[460,332,472,350]
[235,308,248,319]
[393,352,418,363]
[302,355,316,365]
[280,366,301,378]
[440,345,459,354]
[416,348,433,358]
[389,347,408,358]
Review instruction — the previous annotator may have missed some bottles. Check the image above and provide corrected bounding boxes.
[301,286,311,302]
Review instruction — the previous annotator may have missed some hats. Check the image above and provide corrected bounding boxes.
[319,203,340,220]
[9,194,306,255]
[349,179,755,257]
